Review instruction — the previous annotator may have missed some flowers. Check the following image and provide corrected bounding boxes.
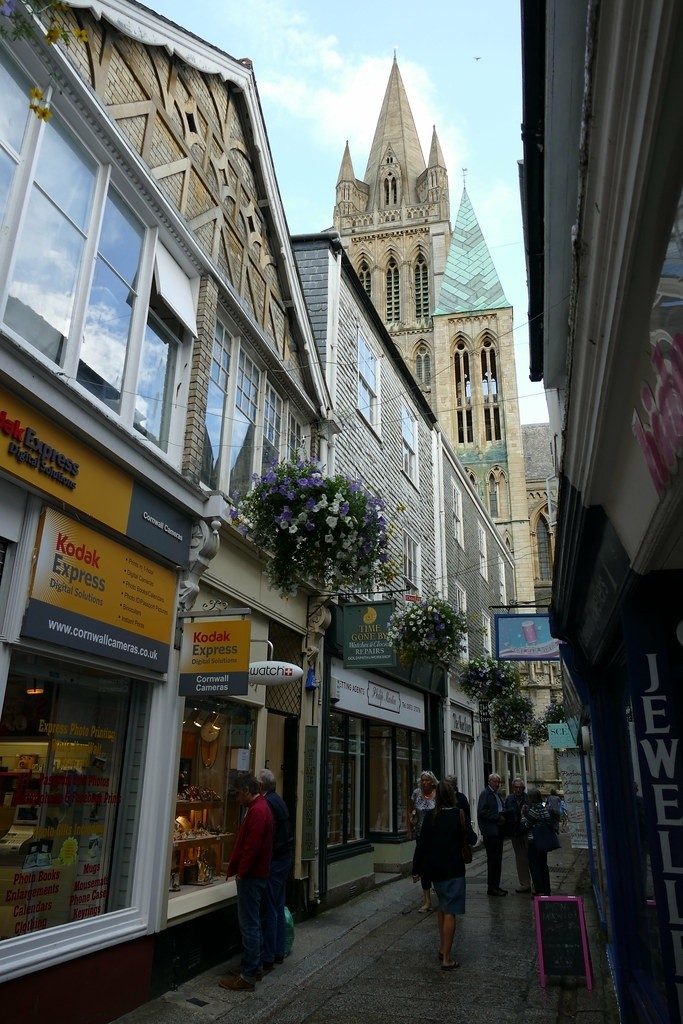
[387,598,490,672]
[227,456,407,600]
[0,0,88,122]
[456,656,566,747]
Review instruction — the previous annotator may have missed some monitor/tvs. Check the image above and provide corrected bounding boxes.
[12,805,41,825]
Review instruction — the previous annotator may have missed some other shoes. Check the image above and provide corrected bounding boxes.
[275,954,284,964]
[229,966,263,981]
[219,974,256,992]
[487,888,508,896]
[418,903,432,913]
[515,885,531,893]
[532,891,551,897]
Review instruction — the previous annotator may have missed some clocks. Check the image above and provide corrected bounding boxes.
[198,713,221,769]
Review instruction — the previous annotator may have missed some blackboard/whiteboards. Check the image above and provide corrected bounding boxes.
[534,895,593,991]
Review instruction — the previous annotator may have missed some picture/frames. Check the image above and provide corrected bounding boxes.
[494,613,568,660]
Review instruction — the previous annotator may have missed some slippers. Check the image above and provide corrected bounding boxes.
[439,952,444,959]
[441,960,459,970]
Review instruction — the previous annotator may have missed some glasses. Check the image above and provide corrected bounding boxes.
[235,791,241,797]
[449,776,456,780]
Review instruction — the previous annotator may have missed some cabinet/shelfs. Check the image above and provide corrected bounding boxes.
[172,800,237,850]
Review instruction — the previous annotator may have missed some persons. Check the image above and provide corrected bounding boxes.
[411,770,478,971]
[477,773,553,895]
[545,788,568,834]
[218,768,290,991]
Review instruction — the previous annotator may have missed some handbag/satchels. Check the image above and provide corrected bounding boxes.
[472,830,477,845]
[410,809,418,825]
[460,809,472,864]
